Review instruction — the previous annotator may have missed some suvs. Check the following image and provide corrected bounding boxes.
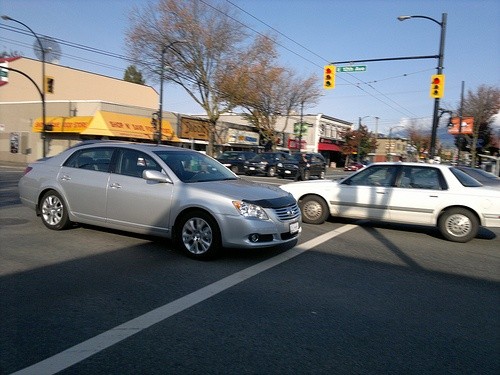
[276,153,327,186]
[243,152,290,177]
[213,151,258,175]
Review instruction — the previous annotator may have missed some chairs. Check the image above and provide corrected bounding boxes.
[162,158,184,180]
[123,157,147,177]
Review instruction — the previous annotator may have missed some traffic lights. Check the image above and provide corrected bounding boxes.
[45,74,55,94]
[46,125,53,131]
[324,65,336,88]
[430,75,445,99]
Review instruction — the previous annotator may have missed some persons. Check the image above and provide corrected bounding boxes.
[294,153,307,182]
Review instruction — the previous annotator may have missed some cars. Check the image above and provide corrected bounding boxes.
[344,162,363,171]
[18,142,302,261]
[278,162,500,243]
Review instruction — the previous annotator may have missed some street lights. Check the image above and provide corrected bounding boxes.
[0,15,47,157]
[151,39,188,144]
[388,125,405,161]
[397,13,454,159]
[357,115,380,163]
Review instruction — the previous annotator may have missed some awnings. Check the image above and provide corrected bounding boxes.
[80,110,180,142]
